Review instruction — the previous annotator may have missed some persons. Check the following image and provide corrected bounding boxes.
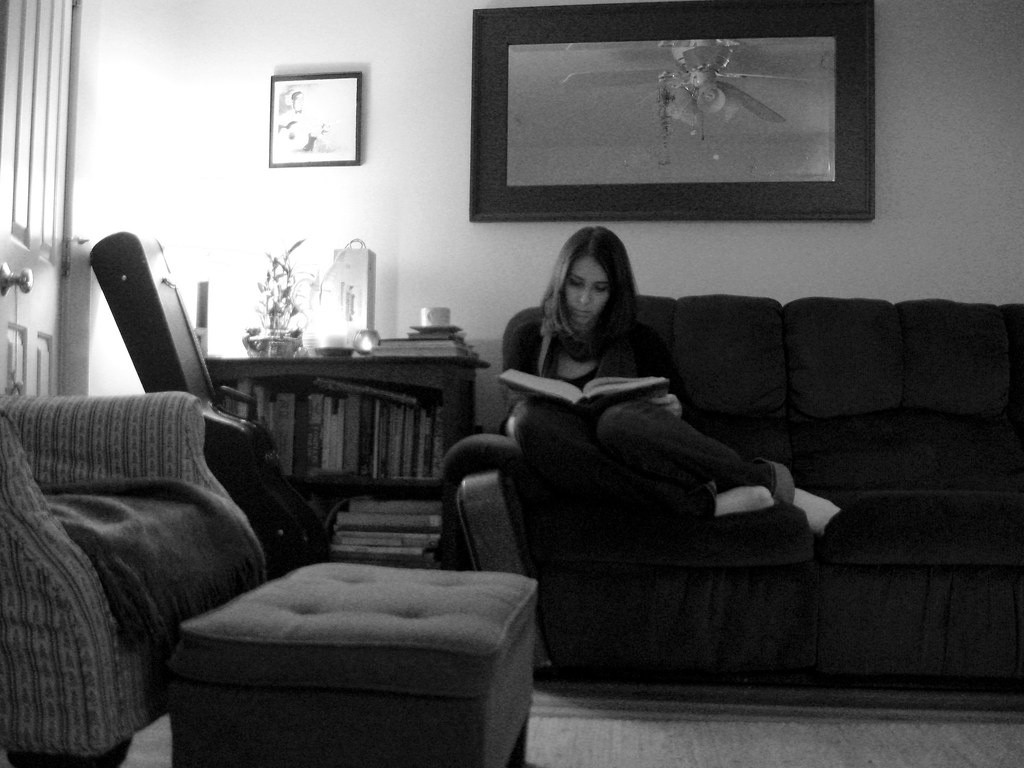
[506,226,841,539]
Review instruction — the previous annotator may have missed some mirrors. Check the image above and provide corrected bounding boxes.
[469,0,875,222]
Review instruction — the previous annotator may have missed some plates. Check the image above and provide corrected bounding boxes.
[315,347,354,358]
[410,325,462,333]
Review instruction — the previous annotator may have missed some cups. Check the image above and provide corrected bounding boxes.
[353,329,380,356]
[421,306,450,326]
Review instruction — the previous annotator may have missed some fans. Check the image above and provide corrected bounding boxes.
[562,42,812,123]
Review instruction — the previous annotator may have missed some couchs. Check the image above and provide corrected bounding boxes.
[440,295,1024,690]
[0,382,264,768]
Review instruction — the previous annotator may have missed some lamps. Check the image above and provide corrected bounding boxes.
[664,84,727,125]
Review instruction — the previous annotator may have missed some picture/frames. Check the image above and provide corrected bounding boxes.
[269,71,362,167]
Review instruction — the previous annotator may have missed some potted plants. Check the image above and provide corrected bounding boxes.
[242,239,317,357]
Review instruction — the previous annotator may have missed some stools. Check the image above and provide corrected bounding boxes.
[167,560,540,768]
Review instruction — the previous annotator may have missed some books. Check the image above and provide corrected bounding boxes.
[496,368,670,415]
[224,333,480,569]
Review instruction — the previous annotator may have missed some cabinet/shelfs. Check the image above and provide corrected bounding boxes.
[207,353,490,567]
